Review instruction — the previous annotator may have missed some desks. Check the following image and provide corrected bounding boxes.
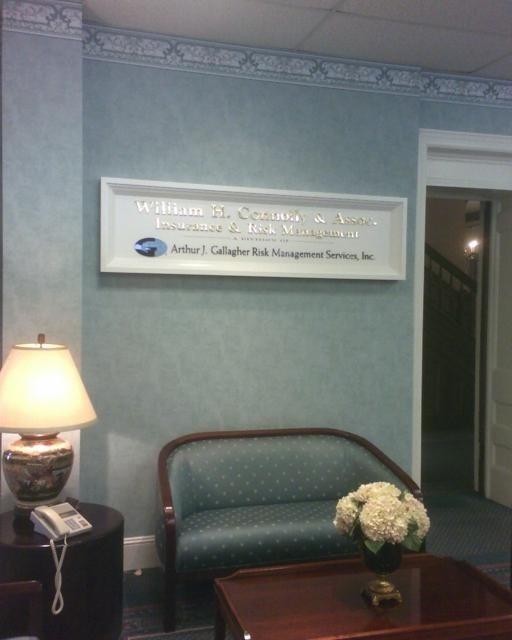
[0,504,126,639]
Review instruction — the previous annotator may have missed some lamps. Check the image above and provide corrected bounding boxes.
[0,330,98,517]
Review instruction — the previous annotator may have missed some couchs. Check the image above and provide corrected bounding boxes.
[148,428,430,633]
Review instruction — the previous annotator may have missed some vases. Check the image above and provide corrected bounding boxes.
[357,536,406,609]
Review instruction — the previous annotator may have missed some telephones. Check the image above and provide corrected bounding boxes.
[29,502,93,542]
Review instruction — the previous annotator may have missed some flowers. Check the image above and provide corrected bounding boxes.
[329,477,431,561]
